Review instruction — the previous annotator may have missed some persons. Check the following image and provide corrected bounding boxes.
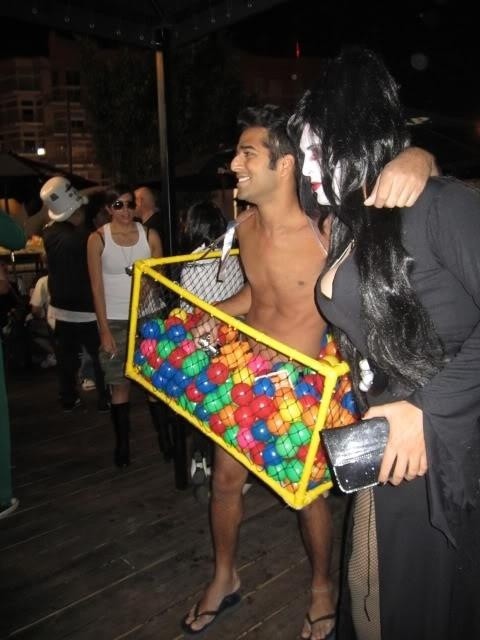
[39,177,178,411]
[184,105,439,639]
[2,204,49,517]
[87,186,174,466]
[170,200,246,493]
[284,53,480,639]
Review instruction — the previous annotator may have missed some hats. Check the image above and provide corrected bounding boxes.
[40,177,87,223]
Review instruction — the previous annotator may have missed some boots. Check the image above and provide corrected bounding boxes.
[111,402,137,467]
[148,400,174,464]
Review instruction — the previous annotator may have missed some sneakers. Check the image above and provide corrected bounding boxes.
[63,398,83,412]
[98,400,112,414]
[41,352,58,369]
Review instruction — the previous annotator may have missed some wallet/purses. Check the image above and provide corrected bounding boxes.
[318,416,394,495]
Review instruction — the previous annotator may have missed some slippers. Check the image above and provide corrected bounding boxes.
[300,603,336,639]
[181,581,242,634]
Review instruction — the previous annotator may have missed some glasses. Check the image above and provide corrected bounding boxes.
[113,199,137,210]
